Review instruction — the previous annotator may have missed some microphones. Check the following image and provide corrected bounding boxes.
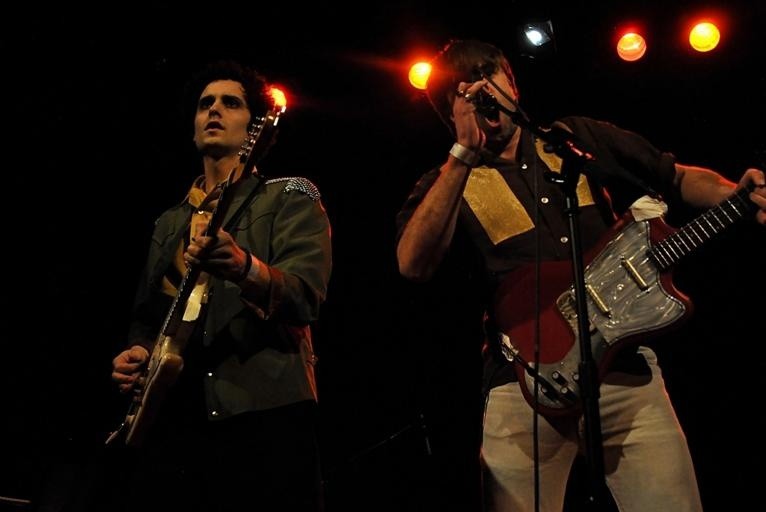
[475,87,500,123]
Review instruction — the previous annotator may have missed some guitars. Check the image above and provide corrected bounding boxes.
[495,178,758,418]
[104,105,282,446]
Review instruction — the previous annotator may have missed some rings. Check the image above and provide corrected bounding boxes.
[464,93,475,101]
[457,89,465,98]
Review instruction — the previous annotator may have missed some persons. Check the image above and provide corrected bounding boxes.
[388,36,765,512]
[109,63,339,511]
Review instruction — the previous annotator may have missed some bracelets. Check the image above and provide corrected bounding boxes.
[237,246,253,285]
[448,144,482,168]
